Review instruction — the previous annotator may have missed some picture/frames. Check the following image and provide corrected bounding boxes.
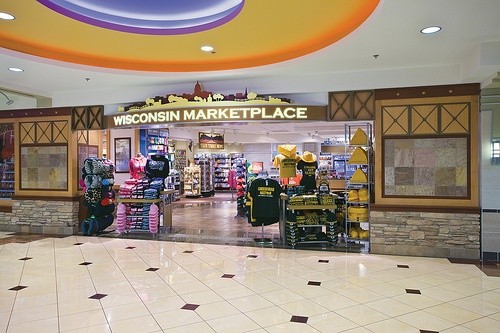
[114,137,131,173]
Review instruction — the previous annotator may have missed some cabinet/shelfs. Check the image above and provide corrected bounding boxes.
[140,128,369,201]
[114,189,174,238]
[344,123,375,249]
[0,154,15,199]
[279,193,338,248]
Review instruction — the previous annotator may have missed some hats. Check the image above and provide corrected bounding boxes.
[299,151,317,163]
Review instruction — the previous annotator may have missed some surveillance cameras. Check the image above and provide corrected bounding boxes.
[6,100,14,105]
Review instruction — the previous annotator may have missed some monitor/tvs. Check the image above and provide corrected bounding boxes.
[198,132,224,149]
